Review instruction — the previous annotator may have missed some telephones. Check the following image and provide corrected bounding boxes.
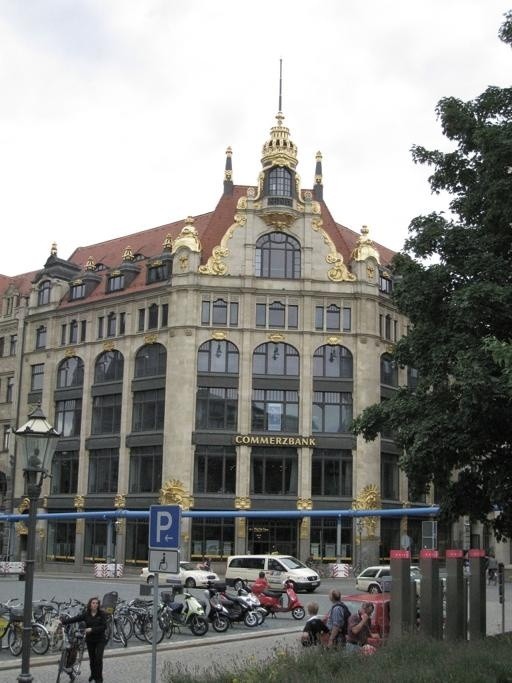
[359,609,368,623]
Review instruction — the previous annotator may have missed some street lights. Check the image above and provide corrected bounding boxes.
[8,402,64,682]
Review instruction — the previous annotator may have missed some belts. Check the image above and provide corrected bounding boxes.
[348,640,364,647]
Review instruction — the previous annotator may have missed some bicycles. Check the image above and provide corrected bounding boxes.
[486,567,498,587]
[48,619,87,682]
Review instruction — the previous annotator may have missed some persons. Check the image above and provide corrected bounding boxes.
[301,603,331,647]
[325,588,351,651]
[346,601,381,652]
[485,553,499,580]
[56,597,109,683]
[28,447,42,483]
[256,571,270,587]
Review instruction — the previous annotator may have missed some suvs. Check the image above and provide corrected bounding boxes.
[354,564,445,598]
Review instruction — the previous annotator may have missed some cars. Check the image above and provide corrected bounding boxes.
[303,588,463,652]
[140,561,218,587]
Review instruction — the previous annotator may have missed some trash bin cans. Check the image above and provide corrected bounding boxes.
[172,584,183,595]
[489,568,497,580]
[140,584,154,595]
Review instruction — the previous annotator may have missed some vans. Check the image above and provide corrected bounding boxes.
[224,552,321,594]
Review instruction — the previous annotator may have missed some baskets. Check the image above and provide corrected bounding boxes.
[32,603,42,616]
[161,591,174,603]
[10,606,24,621]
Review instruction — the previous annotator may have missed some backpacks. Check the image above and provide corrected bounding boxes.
[331,602,351,635]
